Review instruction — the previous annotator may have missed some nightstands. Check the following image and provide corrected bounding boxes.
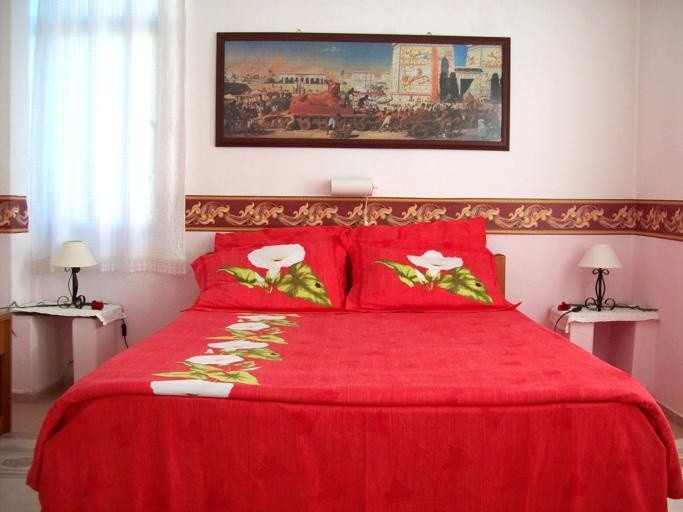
[550,308,659,410]
[11,304,121,403]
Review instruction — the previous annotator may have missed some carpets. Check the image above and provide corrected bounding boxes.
[0,438,40,512]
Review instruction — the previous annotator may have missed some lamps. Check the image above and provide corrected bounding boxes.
[576,243,623,312]
[49,240,96,310]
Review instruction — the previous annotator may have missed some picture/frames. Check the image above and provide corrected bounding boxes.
[215,32,510,150]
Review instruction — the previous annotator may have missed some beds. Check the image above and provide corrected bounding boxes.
[24,252,680,512]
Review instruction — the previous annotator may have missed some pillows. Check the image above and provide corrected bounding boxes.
[179,244,347,313]
[345,250,525,311]
[340,216,488,265]
[213,227,354,261]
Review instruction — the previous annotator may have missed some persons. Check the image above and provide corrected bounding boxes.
[224,42,501,142]
